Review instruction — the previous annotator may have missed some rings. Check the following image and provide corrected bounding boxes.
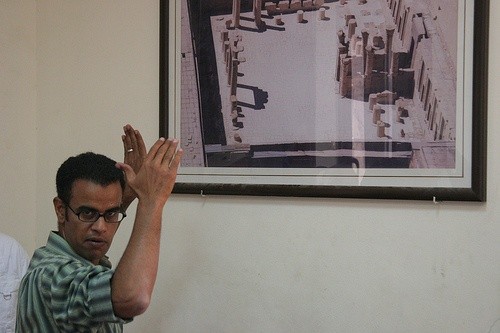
[127,149,133,153]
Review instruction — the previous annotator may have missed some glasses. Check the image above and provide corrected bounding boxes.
[63,200,127,223]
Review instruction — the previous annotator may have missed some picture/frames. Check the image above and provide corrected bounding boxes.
[158,0,490,203]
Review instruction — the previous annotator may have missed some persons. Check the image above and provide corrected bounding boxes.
[14,124,184,333]
[0,232,32,333]
